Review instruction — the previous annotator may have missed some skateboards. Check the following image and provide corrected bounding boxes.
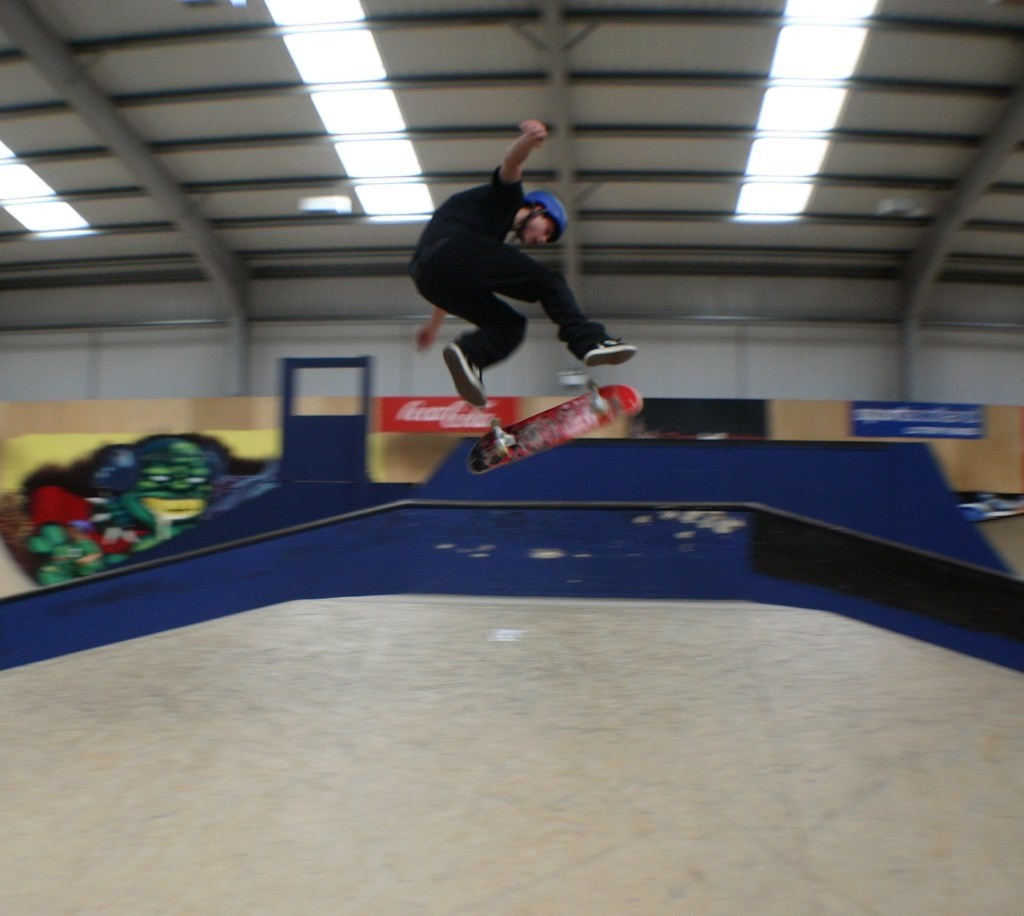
[461,380,645,478]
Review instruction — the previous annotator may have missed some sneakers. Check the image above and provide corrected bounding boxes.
[443,338,487,406]
[580,338,637,366]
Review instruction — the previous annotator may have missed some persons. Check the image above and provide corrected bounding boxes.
[409,120,637,408]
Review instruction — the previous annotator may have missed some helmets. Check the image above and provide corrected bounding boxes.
[524,191,568,243]
